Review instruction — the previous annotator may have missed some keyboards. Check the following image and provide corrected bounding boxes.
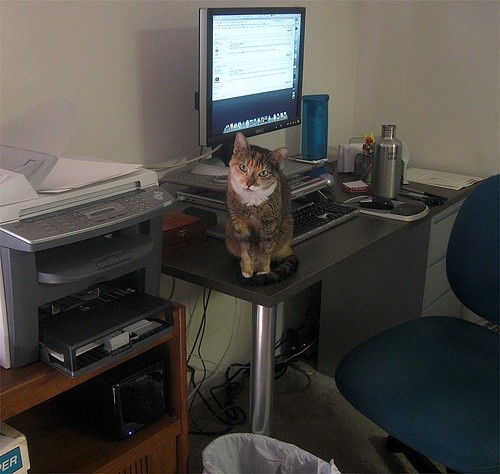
[292,199,359,247]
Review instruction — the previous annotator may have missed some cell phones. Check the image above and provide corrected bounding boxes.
[288,153,328,165]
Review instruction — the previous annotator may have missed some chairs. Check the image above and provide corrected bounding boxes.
[334,172,500,472]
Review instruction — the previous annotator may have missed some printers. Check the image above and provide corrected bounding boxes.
[4,170,180,379]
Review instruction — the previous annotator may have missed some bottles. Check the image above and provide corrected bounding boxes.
[372,124,403,200]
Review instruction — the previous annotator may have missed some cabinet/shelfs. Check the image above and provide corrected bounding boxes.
[0,301,191,472]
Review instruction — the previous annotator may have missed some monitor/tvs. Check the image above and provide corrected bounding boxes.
[191,6,306,178]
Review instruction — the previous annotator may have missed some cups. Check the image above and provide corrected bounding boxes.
[302,93,330,160]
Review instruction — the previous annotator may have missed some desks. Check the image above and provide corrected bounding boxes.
[161,166,486,436]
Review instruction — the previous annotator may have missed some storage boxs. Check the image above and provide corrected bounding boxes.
[163,211,208,261]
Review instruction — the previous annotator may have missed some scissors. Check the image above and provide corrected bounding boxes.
[399,192,445,207]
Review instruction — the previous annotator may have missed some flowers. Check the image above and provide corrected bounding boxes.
[361,131,376,155]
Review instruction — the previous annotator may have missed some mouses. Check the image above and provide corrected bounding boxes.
[357,195,393,210]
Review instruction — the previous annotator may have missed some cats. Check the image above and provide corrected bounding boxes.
[224,133,299,285]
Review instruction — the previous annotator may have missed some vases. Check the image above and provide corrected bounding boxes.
[361,152,375,186]
